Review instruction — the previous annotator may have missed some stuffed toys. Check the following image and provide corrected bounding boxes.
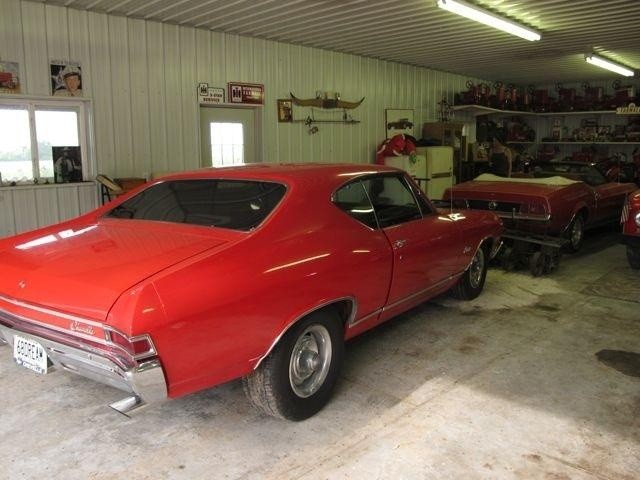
[375,133,417,165]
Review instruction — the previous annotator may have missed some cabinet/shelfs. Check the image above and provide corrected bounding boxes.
[448,103,640,145]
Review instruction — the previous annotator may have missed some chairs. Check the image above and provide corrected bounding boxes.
[101,177,147,208]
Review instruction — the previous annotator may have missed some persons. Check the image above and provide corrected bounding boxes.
[488,134,513,177]
[53,65,81,96]
[55,147,81,182]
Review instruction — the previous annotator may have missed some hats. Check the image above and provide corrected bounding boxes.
[61,65,81,77]
[62,148,70,151]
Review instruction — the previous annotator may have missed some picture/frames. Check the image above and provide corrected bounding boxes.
[385,108,414,140]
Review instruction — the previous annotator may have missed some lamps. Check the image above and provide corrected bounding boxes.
[585,54,635,80]
[436,0,541,42]
